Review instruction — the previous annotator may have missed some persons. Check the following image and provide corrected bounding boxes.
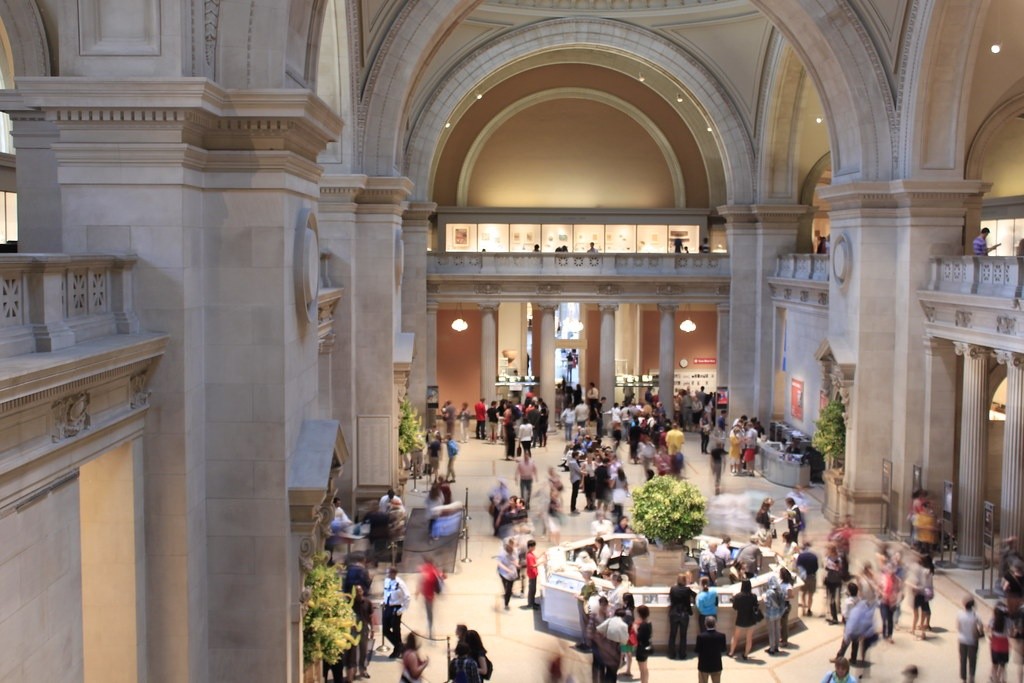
[972,227,996,256]
[320,339,1024,682]
[815,234,830,254]
[672,234,711,254]
[1016,238,1024,255]
[586,241,599,254]
[531,244,540,252]
[555,246,568,252]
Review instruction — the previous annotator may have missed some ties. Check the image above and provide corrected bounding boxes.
[386,583,391,608]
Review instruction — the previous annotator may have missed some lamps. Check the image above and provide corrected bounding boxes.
[679,303,696,333]
[451,301,469,332]
[562,303,584,333]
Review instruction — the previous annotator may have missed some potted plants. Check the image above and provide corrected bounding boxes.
[627,474,711,550]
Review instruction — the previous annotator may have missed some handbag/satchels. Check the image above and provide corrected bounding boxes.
[644,644,655,656]
[921,585,935,602]
[864,633,878,649]
[973,613,985,638]
[627,627,638,648]
[517,443,521,456]
[771,527,778,539]
[433,575,445,596]
[442,415,448,421]
[481,659,493,682]
[752,606,764,622]
[791,522,806,534]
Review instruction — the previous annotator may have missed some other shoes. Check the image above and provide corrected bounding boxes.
[576,643,590,653]
[764,636,790,655]
[1012,629,1023,638]
[527,601,540,610]
[803,608,839,625]
[830,657,857,665]
[345,646,403,683]
[962,676,1008,683]
[442,478,456,484]
[622,672,633,678]
[520,589,525,600]
